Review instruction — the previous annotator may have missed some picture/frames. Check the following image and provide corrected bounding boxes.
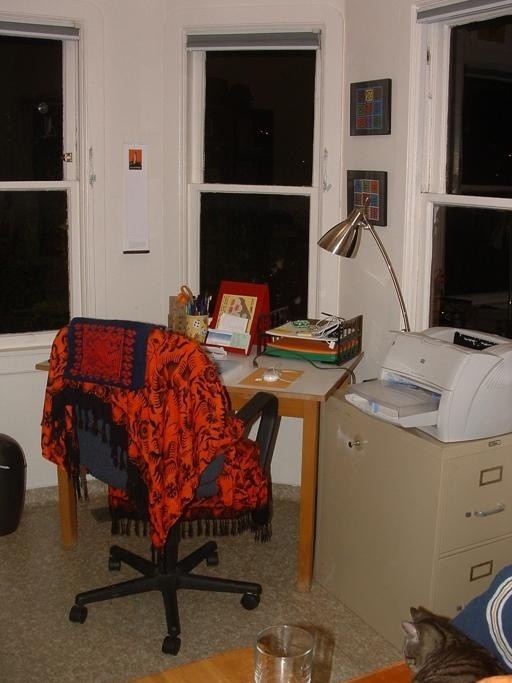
[348,76,392,136]
[346,168,389,227]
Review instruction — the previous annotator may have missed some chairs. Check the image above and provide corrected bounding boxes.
[41,316,280,656]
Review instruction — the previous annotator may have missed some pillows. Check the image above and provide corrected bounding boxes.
[450,564,511,671]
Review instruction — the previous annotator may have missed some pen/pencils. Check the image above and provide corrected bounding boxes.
[185,291,212,316]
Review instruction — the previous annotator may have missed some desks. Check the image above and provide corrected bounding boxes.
[35,338,365,595]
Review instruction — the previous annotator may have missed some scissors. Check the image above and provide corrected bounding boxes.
[178,285,197,309]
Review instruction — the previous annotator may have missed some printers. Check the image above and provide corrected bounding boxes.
[345,327,512,443]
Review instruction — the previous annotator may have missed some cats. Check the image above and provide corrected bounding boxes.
[400,606,511,683]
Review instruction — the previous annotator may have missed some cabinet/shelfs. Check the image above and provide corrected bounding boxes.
[313,394,511,656]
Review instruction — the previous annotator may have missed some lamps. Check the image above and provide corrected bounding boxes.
[316,193,411,331]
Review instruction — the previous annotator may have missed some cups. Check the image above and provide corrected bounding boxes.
[185,315,208,344]
[255,624,314,683]
